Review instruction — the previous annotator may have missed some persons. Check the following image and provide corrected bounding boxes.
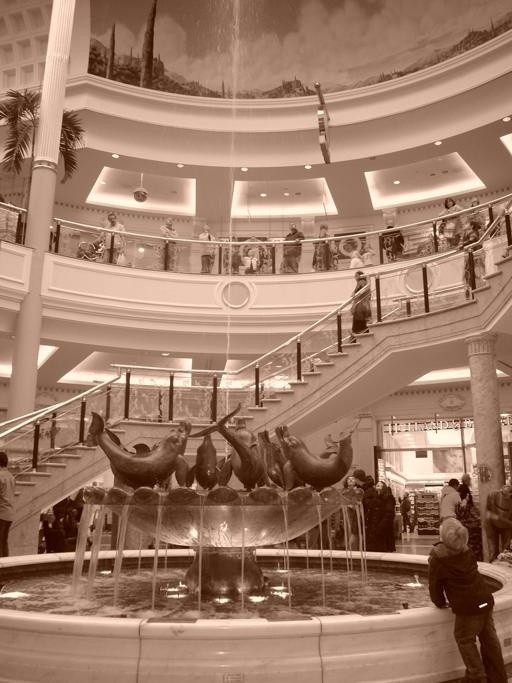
[199,225,217,274]
[161,218,178,270]
[352,272,371,343]
[458,474,474,508]
[345,470,396,553]
[439,197,465,250]
[464,221,484,302]
[1,451,15,555]
[429,518,508,683]
[92,214,128,264]
[398,493,414,532]
[282,221,304,273]
[381,219,404,262]
[440,478,469,520]
[311,224,331,271]
[238,249,259,274]
[468,198,486,243]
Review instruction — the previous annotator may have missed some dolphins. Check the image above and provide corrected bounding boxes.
[81,401,353,494]
